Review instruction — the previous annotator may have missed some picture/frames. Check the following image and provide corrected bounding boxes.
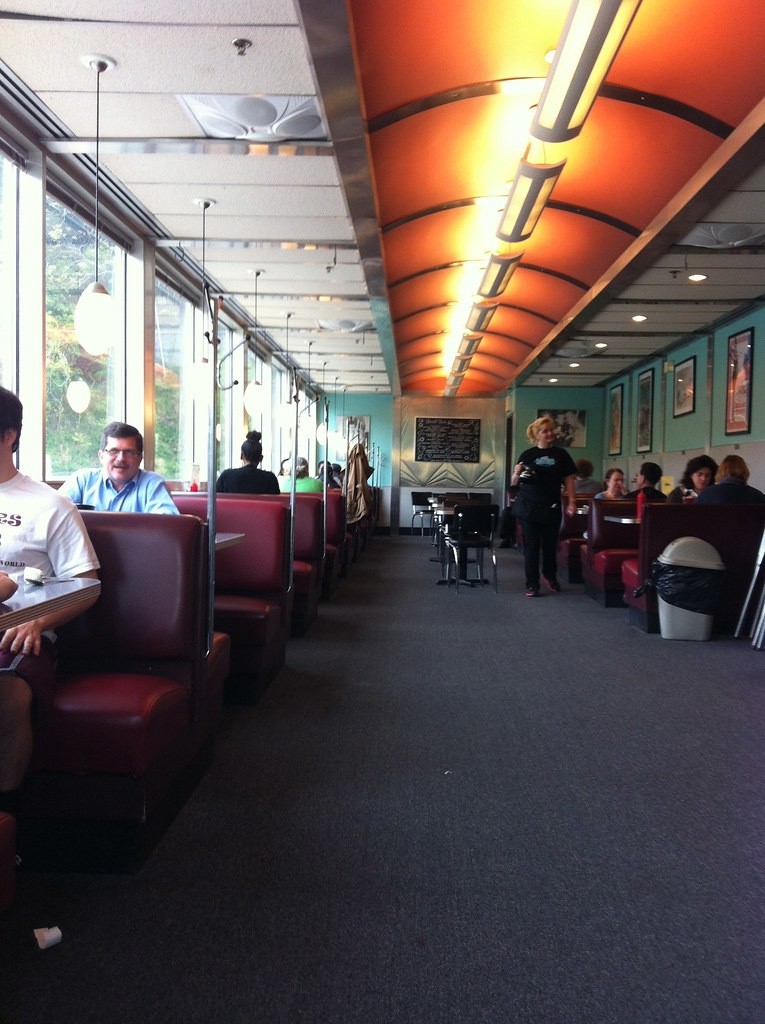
[725,326,756,436]
[636,368,654,454]
[537,408,588,448]
[608,383,624,457]
[672,355,695,419]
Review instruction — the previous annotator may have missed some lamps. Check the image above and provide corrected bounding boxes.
[68,374,91,414]
[439,0,645,398]
[70,55,348,456]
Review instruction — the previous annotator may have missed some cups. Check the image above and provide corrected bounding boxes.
[682,496,696,503]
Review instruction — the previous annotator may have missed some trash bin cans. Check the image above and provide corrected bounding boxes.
[654,537,724,642]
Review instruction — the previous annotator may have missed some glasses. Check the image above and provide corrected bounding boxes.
[609,479,625,482]
[104,447,140,457]
[635,472,642,477]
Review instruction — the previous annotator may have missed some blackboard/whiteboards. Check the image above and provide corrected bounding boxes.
[415,416,481,464]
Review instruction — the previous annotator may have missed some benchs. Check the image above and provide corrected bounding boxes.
[509,486,765,634]
[0,489,376,909]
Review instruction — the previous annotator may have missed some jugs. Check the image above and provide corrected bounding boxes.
[520,463,536,484]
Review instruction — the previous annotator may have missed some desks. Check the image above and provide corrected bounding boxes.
[575,507,588,514]
[411,490,499,595]
[435,508,495,588]
[0,575,101,633]
[215,532,247,551]
[604,514,641,524]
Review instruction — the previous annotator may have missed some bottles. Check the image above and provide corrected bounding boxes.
[191,480,198,492]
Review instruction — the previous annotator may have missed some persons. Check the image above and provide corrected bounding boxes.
[277,457,345,493]
[56,421,180,515]
[216,431,280,495]
[666,455,718,502]
[511,414,578,597]
[1,384,101,822]
[622,462,668,499]
[574,459,601,492]
[695,455,765,506]
[593,468,631,499]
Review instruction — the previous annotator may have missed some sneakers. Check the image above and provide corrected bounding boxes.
[527,584,540,596]
[541,573,560,592]
